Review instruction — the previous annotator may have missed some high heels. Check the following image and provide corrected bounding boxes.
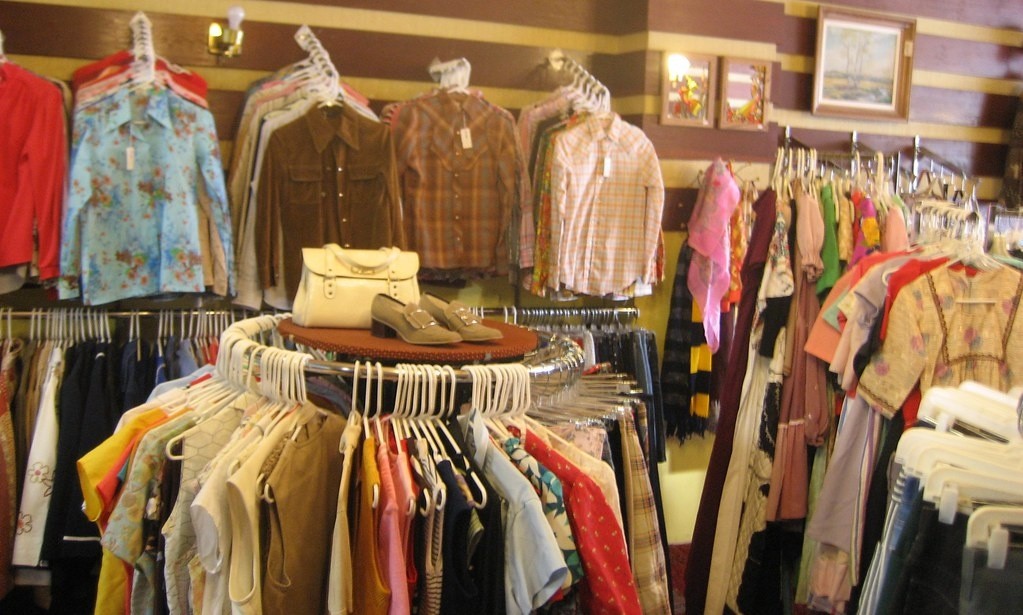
[371,293,462,344]
[419,291,503,341]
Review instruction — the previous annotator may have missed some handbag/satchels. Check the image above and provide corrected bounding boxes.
[291,243,420,328]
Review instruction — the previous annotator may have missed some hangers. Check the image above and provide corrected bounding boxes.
[0,12,1023,516]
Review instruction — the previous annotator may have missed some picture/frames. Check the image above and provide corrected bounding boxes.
[660,49,717,128]
[719,56,773,132]
[812,3,917,124]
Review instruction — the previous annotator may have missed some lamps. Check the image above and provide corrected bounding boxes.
[208,7,245,57]
